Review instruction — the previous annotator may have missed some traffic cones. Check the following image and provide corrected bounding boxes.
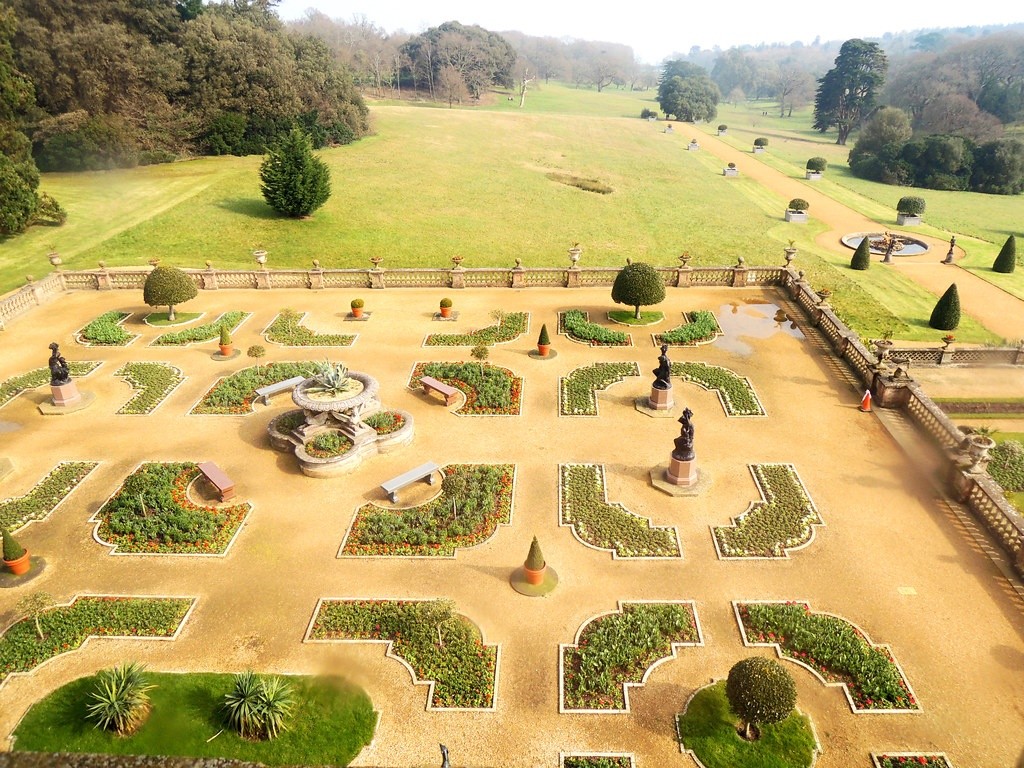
[857,392,872,412]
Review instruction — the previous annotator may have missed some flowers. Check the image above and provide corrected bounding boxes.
[821,288,829,295]
[944,334,954,341]
[569,242,581,251]
[149,256,158,262]
[682,249,690,258]
[679,256,693,269]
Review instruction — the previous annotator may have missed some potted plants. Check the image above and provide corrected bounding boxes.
[219,324,233,356]
[874,329,894,354]
[1,530,30,575]
[784,238,798,260]
[351,299,364,317]
[968,422,1001,456]
[440,298,452,317]
[47,244,63,273]
[538,324,551,356]
[524,535,546,585]
[451,254,464,270]
[370,255,383,270]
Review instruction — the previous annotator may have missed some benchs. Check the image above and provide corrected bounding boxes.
[255,375,305,405]
[195,461,235,502]
[420,376,458,406]
[380,461,439,503]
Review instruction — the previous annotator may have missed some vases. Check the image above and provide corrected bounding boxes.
[568,249,582,269]
[254,250,267,262]
[941,338,955,350]
[149,259,161,268]
[816,291,833,305]
